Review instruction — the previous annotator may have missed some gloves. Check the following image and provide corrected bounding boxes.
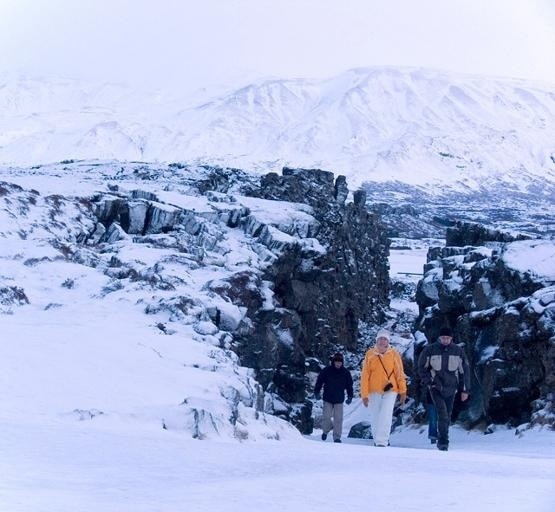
[400,398,405,406]
[315,393,321,400]
[346,395,353,405]
[362,397,369,406]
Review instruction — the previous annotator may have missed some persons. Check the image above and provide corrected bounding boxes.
[418,327,471,451]
[361,337,407,446]
[314,352,353,442]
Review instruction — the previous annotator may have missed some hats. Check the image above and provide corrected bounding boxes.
[375,329,390,345]
[439,325,453,338]
[330,352,344,367]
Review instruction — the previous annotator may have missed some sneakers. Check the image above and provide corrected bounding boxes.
[334,439,341,443]
[431,439,449,451]
[373,442,390,447]
[322,430,330,441]
